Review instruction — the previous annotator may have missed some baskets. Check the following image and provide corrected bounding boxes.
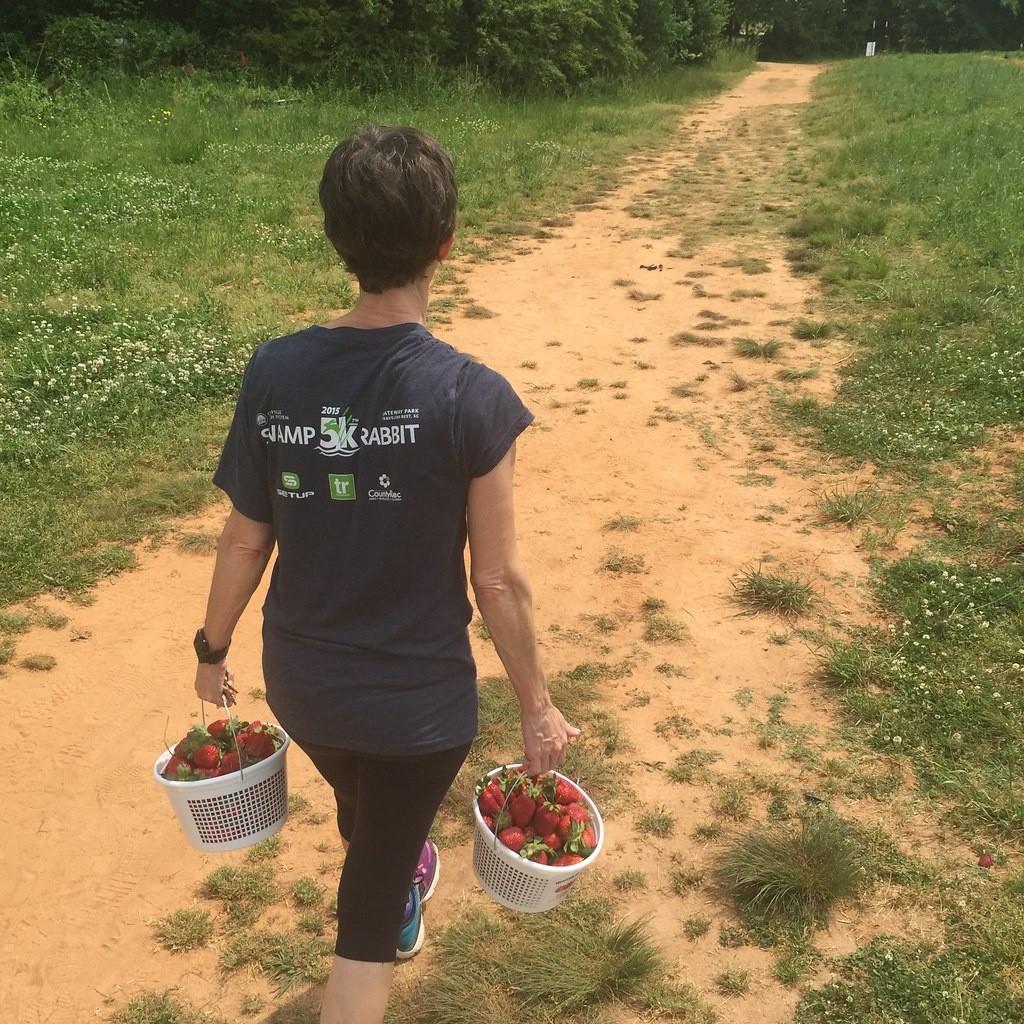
[472,760,603,911]
[155,692,289,850]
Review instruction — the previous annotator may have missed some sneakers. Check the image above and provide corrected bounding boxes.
[389,838,439,960]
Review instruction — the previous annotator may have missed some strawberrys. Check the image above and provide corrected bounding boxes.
[165,719,284,783]
[475,764,595,866]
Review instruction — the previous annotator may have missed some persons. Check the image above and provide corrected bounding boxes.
[188,128,581,1024]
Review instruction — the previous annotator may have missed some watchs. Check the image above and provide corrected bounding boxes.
[192,626,232,666]
